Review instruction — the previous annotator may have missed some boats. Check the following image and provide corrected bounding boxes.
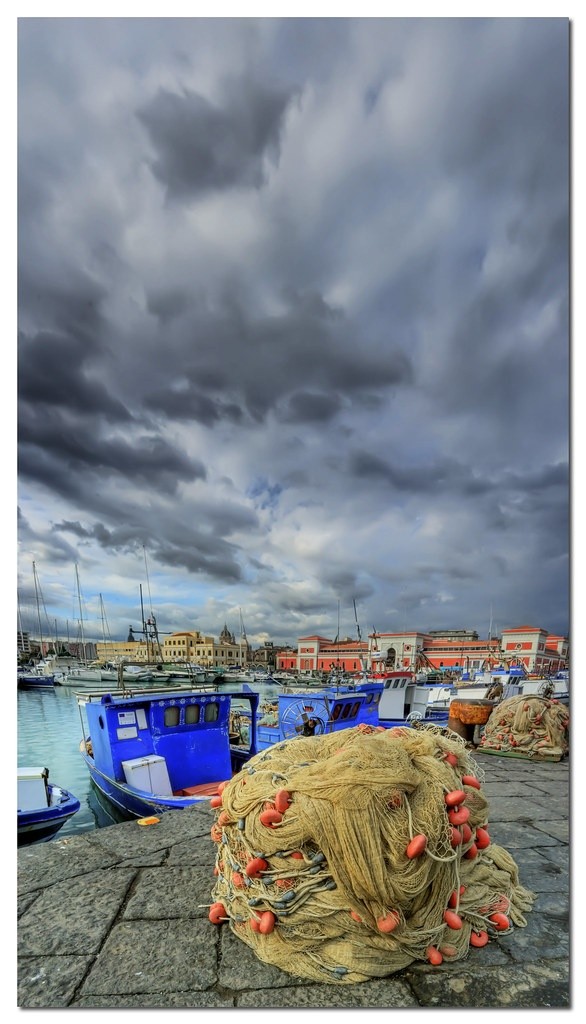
[18,767,81,848]
[72,683,234,822]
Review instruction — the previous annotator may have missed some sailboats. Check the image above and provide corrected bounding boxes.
[17,543,572,764]
